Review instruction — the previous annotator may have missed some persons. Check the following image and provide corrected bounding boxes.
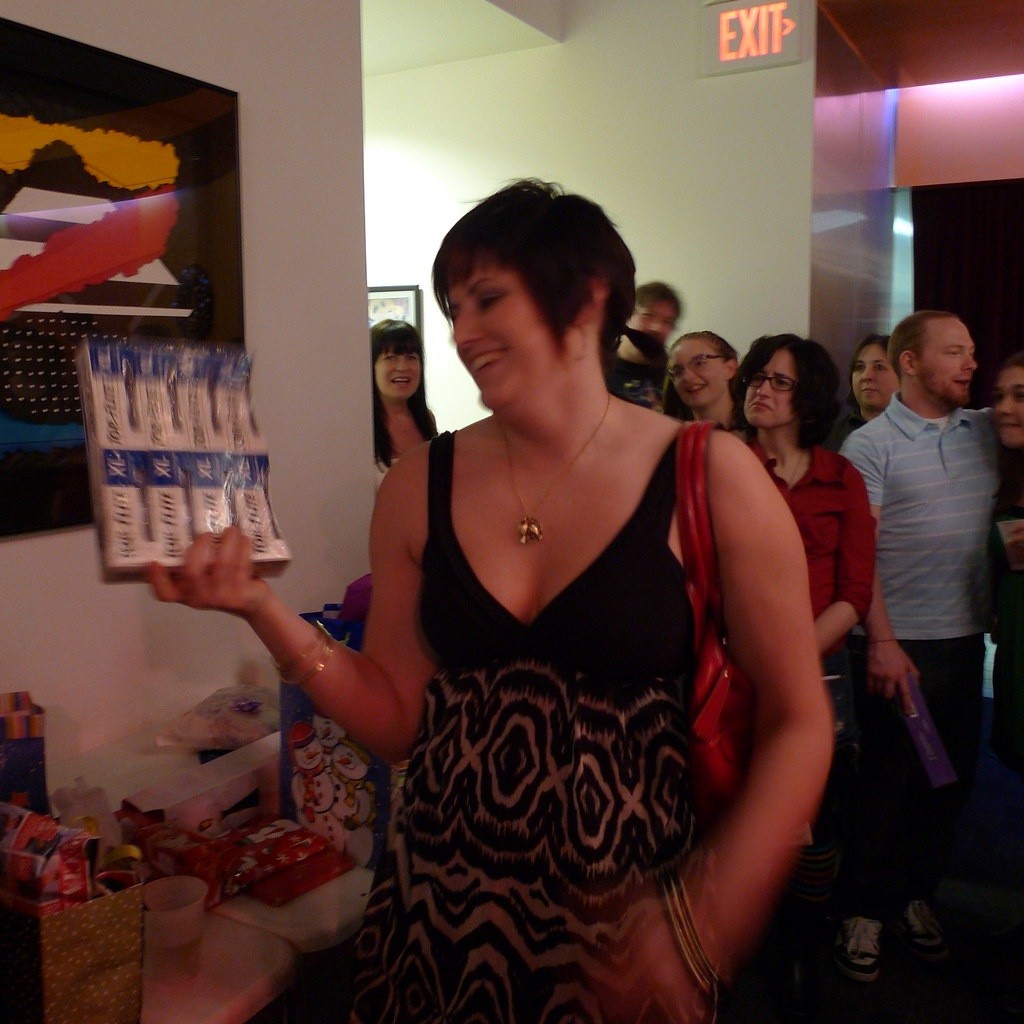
[825,311,1024,1024]
[608,281,683,415]
[669,331,758,437]
[141,177,837,1023]
[359,318,443,488]
[727,333,877,1021]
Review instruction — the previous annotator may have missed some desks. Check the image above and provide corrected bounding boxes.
[141,867,379,1024]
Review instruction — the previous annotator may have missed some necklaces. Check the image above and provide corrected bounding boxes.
[495,392,612,544]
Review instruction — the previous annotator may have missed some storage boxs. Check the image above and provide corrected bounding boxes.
[1,876,141,1023]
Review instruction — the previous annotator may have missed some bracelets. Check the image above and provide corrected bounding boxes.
[277,622,332,682]
[656,866,724,994]
[869,639,898,644]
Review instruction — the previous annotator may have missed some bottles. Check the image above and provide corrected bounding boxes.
[56,776,123,852]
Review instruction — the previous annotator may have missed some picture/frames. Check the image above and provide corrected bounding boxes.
[368,285,420,331]
[1,12,245,539]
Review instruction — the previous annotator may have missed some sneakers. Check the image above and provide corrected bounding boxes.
[834,916,884,983]
[893,900,949,959]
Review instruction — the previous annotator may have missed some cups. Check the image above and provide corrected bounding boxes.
[143,876,208,956]
[995,519,1024,571]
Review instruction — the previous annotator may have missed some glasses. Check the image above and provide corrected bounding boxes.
[742,368,796,392]
[666,353,729,382]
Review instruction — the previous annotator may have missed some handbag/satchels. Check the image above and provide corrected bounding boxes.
[278,604,412,872]
[0,847,146,1024]
